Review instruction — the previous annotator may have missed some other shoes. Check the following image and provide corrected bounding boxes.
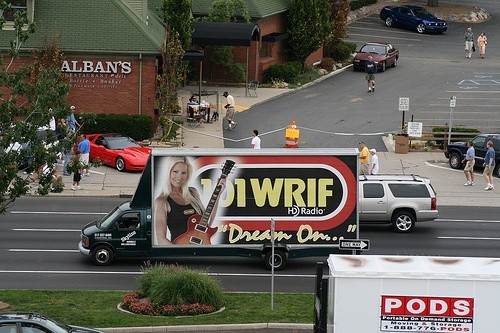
[71,185,75,191]
[464,180,475,186]
[232,122,237,129]
[75,186,84,190]
[479,56,485,59]
[465,55,472,59]
[368,87,376,93]
[485,183,494,191]
[226,126,232,130]
[85,172,90,177]
[63,172,69,176]
[81,173,85,178]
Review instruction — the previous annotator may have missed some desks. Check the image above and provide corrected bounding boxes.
[201,104,216,124]
[192,90,219,113]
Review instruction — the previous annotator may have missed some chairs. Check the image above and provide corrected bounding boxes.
[188,105,206,128]
[248,80,259,97]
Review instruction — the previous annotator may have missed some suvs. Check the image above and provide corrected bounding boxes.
[0,311,105,333]
[444,134,500,178]
[359,173,440,234]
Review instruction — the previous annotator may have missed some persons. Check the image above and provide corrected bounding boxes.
[364,56,376,92]
[71,152,84,190]
[155,156,226,244]
[358,142,379,174]
[464,28,474,59]
[78,134,90,176]
[223,92,238,130]
[477,32,488,59]
[57,106,81,176]
[482,141,495,190]
[189,95,199,112]
[462,140,475,186]
[251,130,261,148]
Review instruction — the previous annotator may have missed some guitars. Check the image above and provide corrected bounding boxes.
[173,160,237,246]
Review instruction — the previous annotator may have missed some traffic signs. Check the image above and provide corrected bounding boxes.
[338,238,371,251]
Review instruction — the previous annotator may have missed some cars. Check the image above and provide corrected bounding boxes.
[353,42,399,72]
[379,5,448,34]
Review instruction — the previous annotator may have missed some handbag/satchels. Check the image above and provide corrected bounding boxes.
[472,45,476,52]
[485,40,487,45]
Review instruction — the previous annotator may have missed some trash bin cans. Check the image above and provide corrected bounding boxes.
[395,134,409,153]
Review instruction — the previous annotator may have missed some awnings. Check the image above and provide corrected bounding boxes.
[190,22,260,96]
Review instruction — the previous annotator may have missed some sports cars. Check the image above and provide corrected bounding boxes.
[73,132,152,172]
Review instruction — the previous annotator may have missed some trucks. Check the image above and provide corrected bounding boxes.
[312,253,500,333]
[77,147,360,271]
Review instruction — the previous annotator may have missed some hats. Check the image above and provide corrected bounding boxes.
[223,91,228,96]
[369,147,376,154]
